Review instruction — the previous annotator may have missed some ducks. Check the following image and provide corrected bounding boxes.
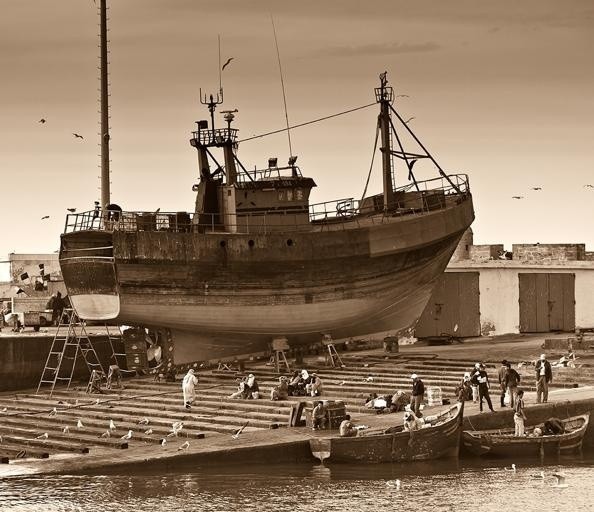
[529,470,569,489]
[503,463,517,472]
[386,479,401,488]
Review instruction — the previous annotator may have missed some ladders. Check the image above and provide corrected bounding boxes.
[105,322,140,379]
[35,307,110,399]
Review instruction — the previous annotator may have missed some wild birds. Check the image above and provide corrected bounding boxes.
[72,133,83,139]
[512,196,522,199]
[39,118,44,123]
[41,215,50,220]
[404,117,414,124]
[222,58,234,71]
[532,187,541,190]
[67,207,77,212]
[37,406,191,452]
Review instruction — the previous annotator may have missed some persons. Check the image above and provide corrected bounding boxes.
[340,414,357,437]
[231,370,323,401]
[458,356,526,436]
[55,291,70,324]
[105,203,122,231]
[312,401,328,430]
[406,405,422,431]
[182,369,199,408]
[46,293,57,308]
[411,373,424,416]
[544,416,565,435]
[535,354,552,404]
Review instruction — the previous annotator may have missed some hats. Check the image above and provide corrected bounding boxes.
[411,374,417,379]
[541,354,546,360]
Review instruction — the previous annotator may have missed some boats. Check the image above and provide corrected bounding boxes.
[311,463,463,480]
[462,405,591,451]
[58,0,479,370]
[311,398,467,466]
